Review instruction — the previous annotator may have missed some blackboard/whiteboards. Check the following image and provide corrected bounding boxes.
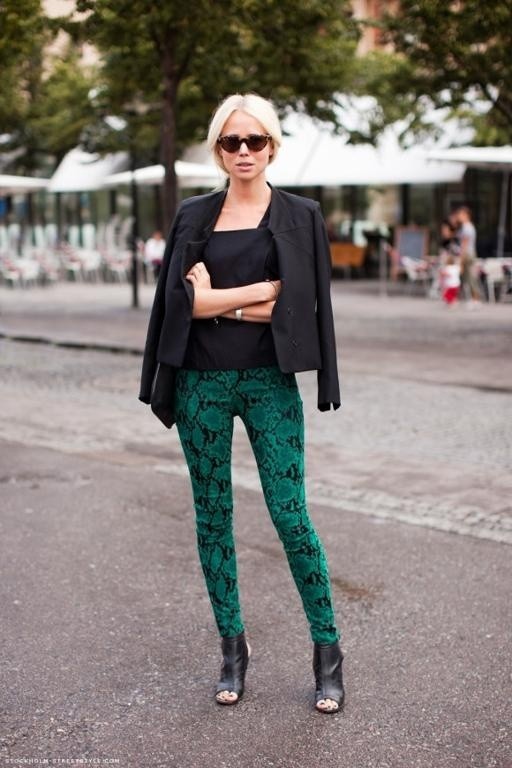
[394,226,429,274]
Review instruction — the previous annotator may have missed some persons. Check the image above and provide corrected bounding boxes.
[138,93,347,714]
[142,230,166,283]
[429,205,483,312]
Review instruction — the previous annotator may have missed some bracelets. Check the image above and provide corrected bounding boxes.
[271,281,279,300]
[235,307,242,322]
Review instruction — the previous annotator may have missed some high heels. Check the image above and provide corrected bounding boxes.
[312,640,345,713]
[215,631,249,705]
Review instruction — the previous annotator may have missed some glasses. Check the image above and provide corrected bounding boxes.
[216,136,272,152]
